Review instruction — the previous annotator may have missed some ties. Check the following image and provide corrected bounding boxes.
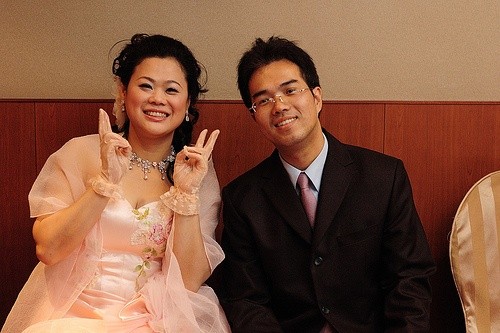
[297,173,317,227]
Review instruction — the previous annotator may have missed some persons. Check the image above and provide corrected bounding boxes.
[0,33,230,333]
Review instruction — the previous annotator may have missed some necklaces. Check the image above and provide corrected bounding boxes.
[115,132,176,181]
[220,37,438,333]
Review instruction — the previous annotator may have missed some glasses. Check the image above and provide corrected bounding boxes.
[248,86,309,112]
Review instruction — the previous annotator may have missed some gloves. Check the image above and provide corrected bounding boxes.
[90,107,133,198]
[160,128,220,215]
